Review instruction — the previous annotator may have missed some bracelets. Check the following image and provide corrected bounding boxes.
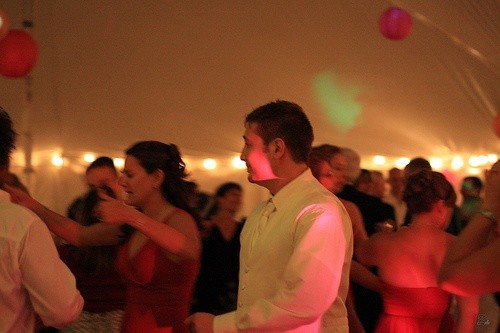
[475,208,497,222]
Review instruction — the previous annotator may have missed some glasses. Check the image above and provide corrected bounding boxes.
[320,170,336,179]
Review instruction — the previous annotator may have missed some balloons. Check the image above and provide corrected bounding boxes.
[378,7,414,40]
[0,12,37,79]
[492,112,500,139]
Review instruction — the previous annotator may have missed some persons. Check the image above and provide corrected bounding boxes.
[184,100,354,332]
[307,144,500,333]
[55,155,248,333]
[5,141,201,333]
[0,106,84,333]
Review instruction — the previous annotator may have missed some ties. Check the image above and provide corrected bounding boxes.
[250,202,274,249]
